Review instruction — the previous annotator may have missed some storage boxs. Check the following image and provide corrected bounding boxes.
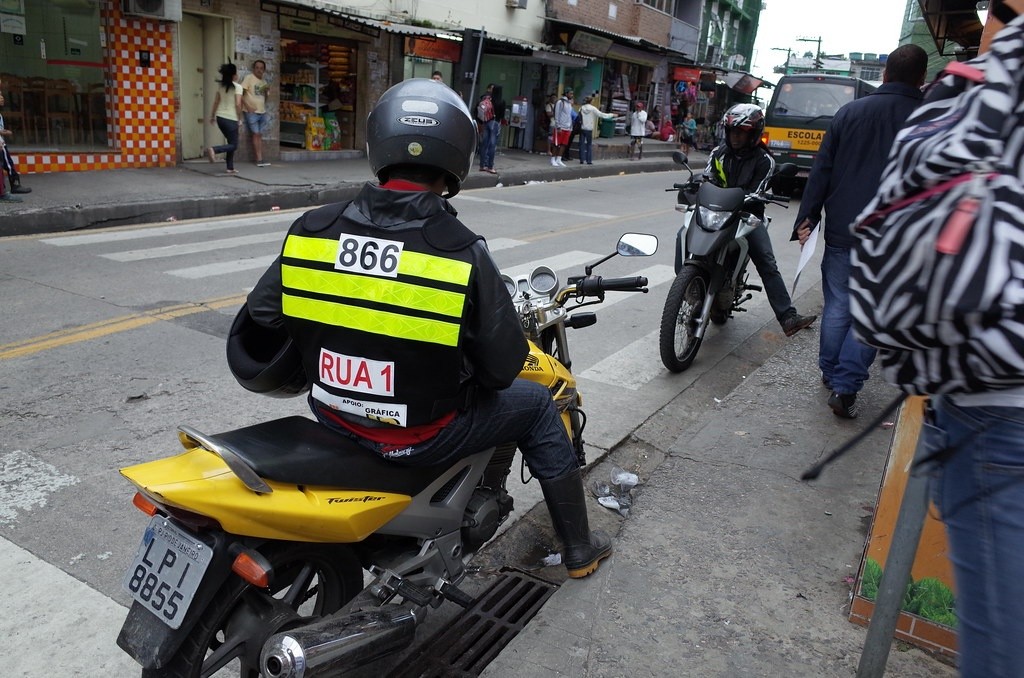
[342,136,354,148]
[340,123,354,136]
[337,111,355,124]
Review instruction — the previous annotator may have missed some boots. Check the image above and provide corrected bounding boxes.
[540,464,612,577]
[551,157,558,166]
[556,156,566,166]
[10,175,32,193]
[630,146,635,160]
[638,146,643,159]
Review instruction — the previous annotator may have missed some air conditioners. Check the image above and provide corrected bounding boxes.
[510,0,527,8]
[120,0,184,23]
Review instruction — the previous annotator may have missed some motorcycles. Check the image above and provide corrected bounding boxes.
[116,233,658,678]
[660,150,799,374]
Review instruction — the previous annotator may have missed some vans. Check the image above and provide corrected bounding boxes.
[761,74,876,199]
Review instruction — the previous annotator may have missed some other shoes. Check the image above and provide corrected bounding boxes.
[479,166,486,171]
[587,162,591,164]
[1,192,22,203]
[580,161,583,164]
[256,160,264,166]
[263,160,272,166]
[208,147,215,163]
[487,167,497,174]
[227,168,239,173]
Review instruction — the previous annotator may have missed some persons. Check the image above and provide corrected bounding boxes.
[208,63,243,172]
[545,87,617,166]
[0,91,32,204]
[791,43,929,419]
[225,77,612,580]
[432,71,442,82]
[844,7,1023,678]
[241,60,271,166]
[629,101,698,161]
[476,83,507,172]
[682,103,816,336]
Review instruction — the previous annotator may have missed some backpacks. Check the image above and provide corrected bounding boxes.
[478,96,495,122]
[850,14,1024,398]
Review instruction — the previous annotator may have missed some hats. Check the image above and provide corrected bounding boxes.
[561,87,573,96]
[636,102,643,108]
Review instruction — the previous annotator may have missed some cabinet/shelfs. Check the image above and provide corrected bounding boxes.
[281,59,330,150]
[637,84,654,113]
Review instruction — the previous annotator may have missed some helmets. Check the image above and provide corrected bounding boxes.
[366,78,478,198]
[722,103,764,147]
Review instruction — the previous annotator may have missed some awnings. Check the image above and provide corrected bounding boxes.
[538,15,687,56]
[670,62,776,94]
[323,9,595,69]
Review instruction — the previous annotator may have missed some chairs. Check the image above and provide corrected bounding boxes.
[0,70,106,149]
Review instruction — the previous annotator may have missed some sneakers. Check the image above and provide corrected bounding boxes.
[782,314,816,337]
[828,392,858,418]
[821,371,832,390]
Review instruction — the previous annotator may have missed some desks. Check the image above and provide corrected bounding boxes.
[20,87,104,142]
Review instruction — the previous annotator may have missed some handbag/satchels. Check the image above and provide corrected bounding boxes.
[574,108,582,134]
[625,122,631,134]
